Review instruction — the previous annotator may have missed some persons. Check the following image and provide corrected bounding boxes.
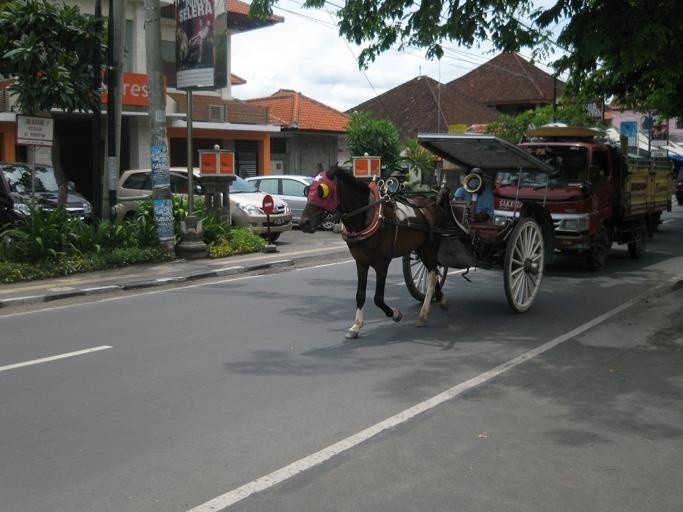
[188,19,212,63]
[452,168,494,225]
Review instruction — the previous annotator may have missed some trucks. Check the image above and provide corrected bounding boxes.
[490,126,675,272]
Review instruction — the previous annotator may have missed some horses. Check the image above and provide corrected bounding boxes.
[298,160,453,339]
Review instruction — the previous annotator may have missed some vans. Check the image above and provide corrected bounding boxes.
[118,165,296,242]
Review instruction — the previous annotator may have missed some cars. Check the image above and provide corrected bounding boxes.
[0,161,93,231]
[245,174,336,231]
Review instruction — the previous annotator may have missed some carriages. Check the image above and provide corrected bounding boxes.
[299,133,557,339]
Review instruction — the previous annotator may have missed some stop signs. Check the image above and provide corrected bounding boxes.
[263,195,273,215]
